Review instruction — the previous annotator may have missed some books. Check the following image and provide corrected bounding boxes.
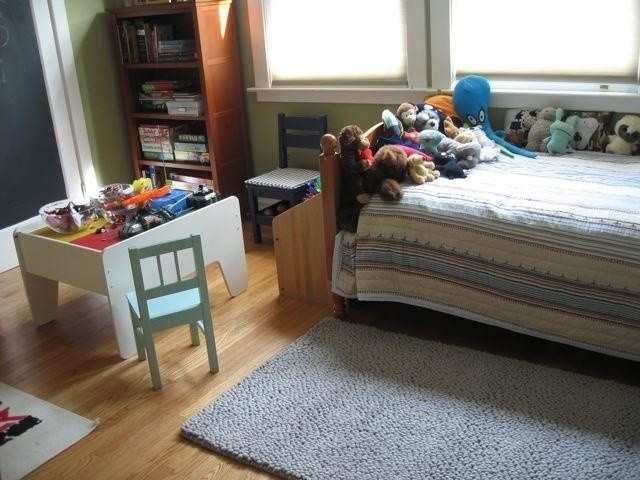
[116,19,195,65]
[137,81,203,101]
[141,166,170,190]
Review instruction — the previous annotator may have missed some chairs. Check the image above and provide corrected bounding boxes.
[244,112,328,244]
[123,231,221,391]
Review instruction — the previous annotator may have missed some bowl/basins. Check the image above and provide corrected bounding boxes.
[99,182,140,224]
[38,196,99,235]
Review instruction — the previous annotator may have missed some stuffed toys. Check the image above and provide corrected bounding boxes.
[336,75,640,204]
[503,107,640,156]
[336,76,539,206]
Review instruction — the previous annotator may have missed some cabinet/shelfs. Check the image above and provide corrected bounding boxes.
[272,193,327,302]
[104,0,257,218]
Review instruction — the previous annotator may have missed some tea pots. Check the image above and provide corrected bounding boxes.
[190,184,217,207]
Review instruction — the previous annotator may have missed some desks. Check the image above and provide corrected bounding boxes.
[12,193,249,359]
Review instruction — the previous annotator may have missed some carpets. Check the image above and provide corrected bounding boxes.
[180,312,638,478]
[1,380,100,480]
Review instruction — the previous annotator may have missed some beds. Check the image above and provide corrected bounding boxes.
[317,101,639,362]
